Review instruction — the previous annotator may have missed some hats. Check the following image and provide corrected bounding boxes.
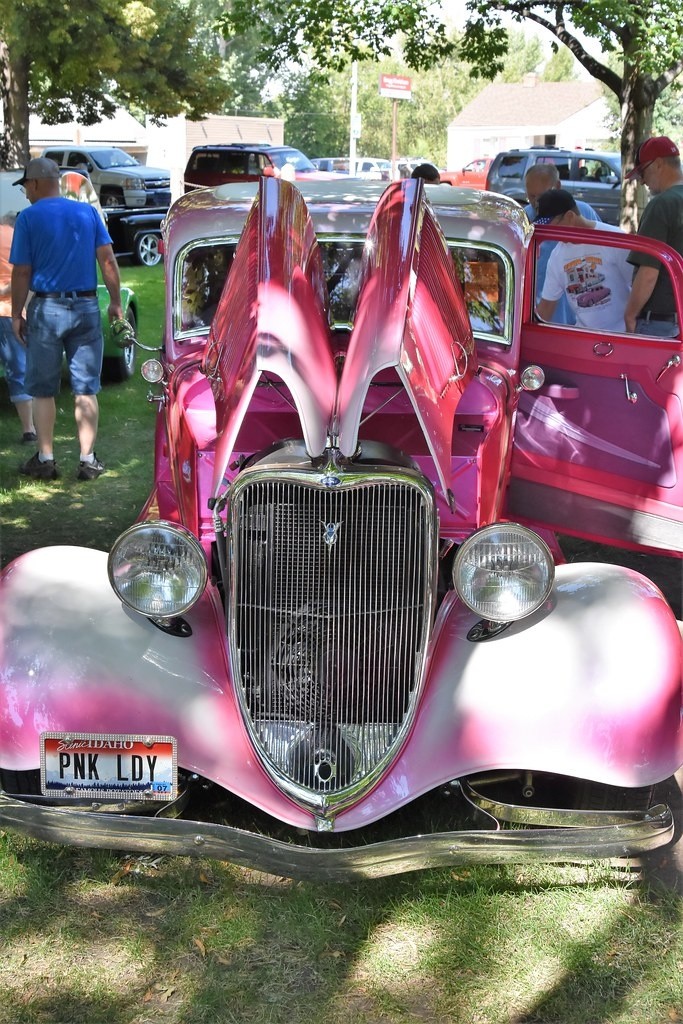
[625,136,680,179]
[12,157,61,186]
[533,189,576,224]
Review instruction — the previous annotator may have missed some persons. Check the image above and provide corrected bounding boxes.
[525,189,635,333]
[518,163,603,324]
[624,138,683,339]
[412,164,440,186]
[12,158,124,482]
[0,225,37,448]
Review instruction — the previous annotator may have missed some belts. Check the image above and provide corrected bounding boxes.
[36,291,96,298]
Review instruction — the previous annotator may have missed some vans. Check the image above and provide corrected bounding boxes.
[486,146,622,225]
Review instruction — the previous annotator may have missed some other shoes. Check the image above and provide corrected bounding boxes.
[24,432,38,446]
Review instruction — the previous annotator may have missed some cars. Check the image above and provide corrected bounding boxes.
[437,158,496,188]
[100,143,438,268]
[0,188,683,882]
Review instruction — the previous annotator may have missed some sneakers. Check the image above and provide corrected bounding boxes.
[77,452,104,480]
[18,451,60,480]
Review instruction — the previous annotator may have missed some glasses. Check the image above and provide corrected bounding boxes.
[20,182,31,194]
[638,160,655,176]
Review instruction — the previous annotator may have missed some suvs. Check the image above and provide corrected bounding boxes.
[40,148,173,205]
